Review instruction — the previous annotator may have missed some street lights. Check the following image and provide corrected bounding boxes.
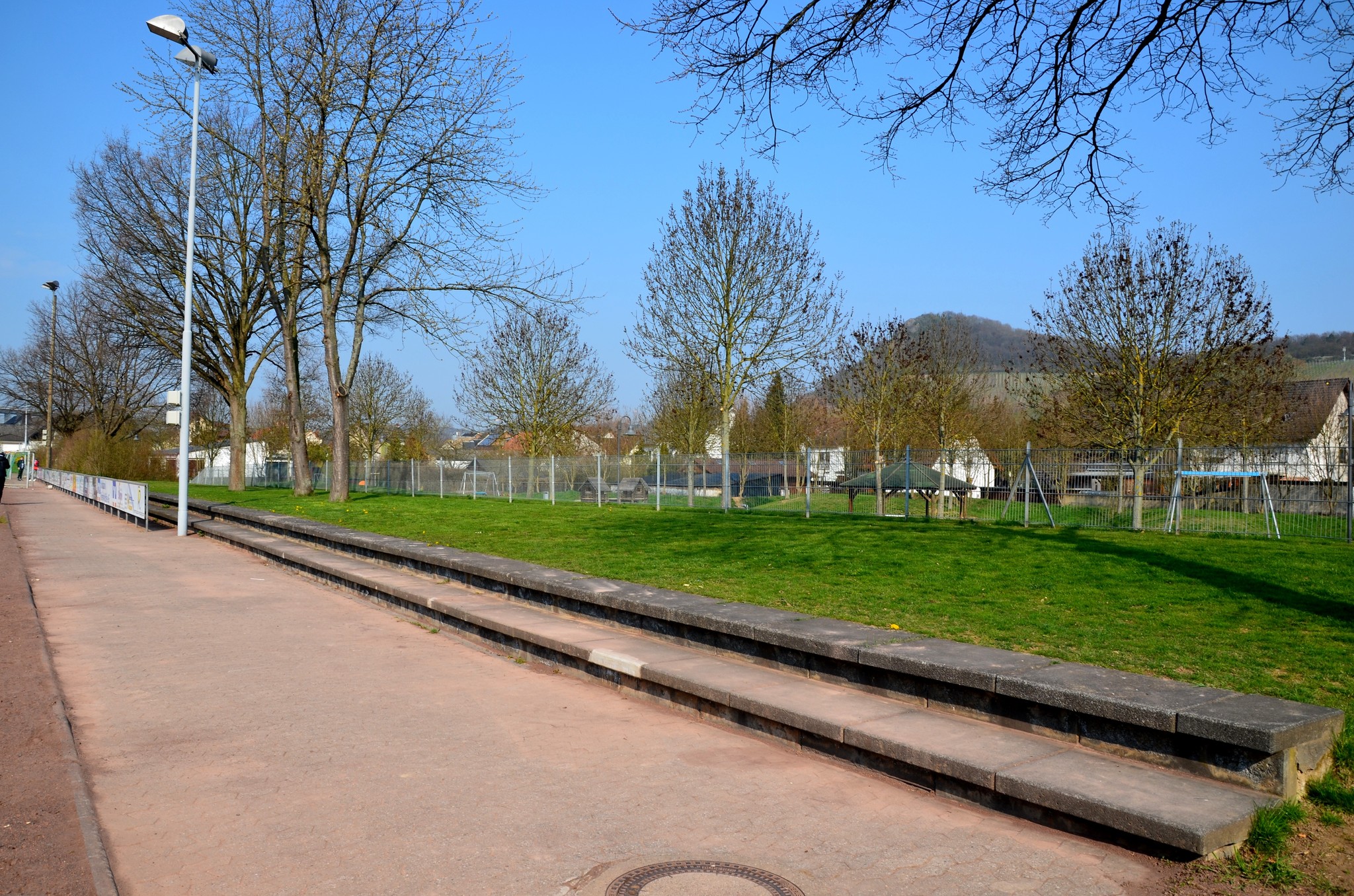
[24,383,31,465]
[372,429,385,487]
[145,14,220,538]
[42,280,60,471]
[1337,380,1354,543]
[128,430,140,464]
[616,416,638,504]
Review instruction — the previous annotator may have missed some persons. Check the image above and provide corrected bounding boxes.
[29,458,39,481]
[0,451,10,504]
[17,456,25,481]
[420,486,423,492]
